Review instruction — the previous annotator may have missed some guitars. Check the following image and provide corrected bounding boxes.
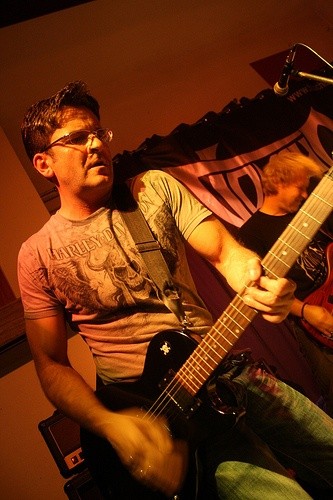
[297,242,333,349]
[77,164,333,500]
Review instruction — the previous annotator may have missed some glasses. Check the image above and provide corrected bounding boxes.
[39,127,113,153]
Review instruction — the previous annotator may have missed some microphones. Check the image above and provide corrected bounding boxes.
[273,44,297,96]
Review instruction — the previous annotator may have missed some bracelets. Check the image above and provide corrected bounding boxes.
[301,303,308,319]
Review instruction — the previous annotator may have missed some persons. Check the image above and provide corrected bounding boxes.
[18,81,333,500]
[236,152,333,339]
